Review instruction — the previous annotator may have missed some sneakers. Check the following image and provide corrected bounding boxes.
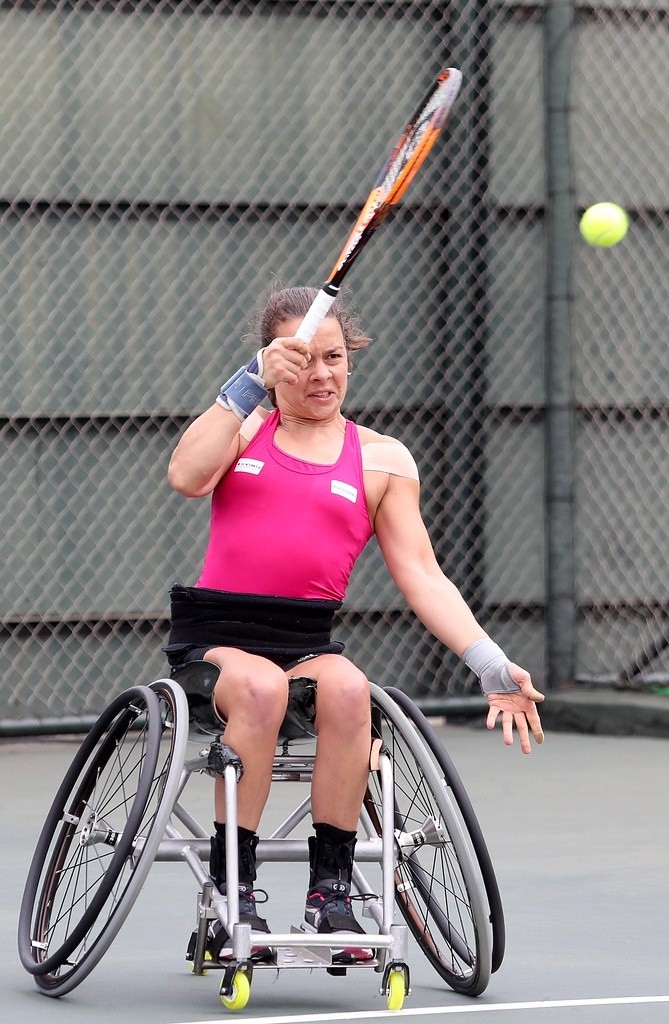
[207,882,278,964]
[304,877,376,961]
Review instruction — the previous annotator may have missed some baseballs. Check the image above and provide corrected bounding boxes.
[579,202,628,245]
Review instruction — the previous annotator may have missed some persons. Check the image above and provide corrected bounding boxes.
[167,286,546,969]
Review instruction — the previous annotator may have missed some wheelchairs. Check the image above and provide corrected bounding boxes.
[17,659,505,1012]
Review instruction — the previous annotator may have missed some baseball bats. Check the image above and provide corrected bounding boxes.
[294,65,463,350]
